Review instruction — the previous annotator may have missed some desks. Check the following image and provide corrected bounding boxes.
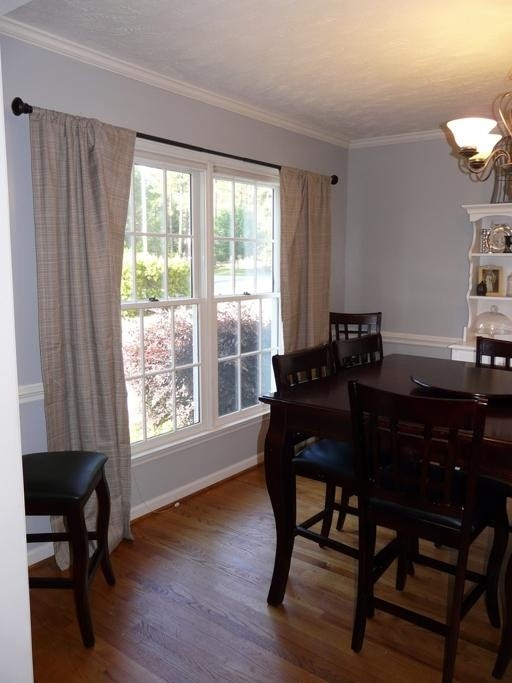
[256,353,511,610]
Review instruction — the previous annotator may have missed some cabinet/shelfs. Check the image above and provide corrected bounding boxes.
[448,201,512,365]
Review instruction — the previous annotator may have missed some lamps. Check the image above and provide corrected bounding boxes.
[444,89,512,184]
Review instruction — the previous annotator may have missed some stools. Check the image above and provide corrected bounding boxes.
[21,451,119,649]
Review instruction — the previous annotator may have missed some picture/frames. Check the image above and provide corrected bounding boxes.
[487,222,512,252]
[477,265,505,296]
